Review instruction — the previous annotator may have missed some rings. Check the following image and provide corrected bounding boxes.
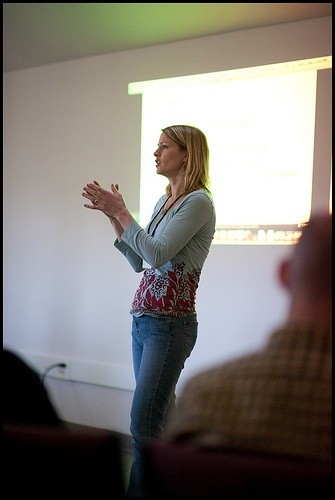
[93,199,98,204]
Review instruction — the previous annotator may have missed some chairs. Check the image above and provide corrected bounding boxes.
[1,423,335,500]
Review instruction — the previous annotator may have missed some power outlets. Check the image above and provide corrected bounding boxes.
[51,357,71,378]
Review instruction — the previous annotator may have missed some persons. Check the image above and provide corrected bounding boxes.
[82,125,216,500]
[159,214,335,458]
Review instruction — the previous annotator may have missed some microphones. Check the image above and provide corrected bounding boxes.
[41,363,66,382]
